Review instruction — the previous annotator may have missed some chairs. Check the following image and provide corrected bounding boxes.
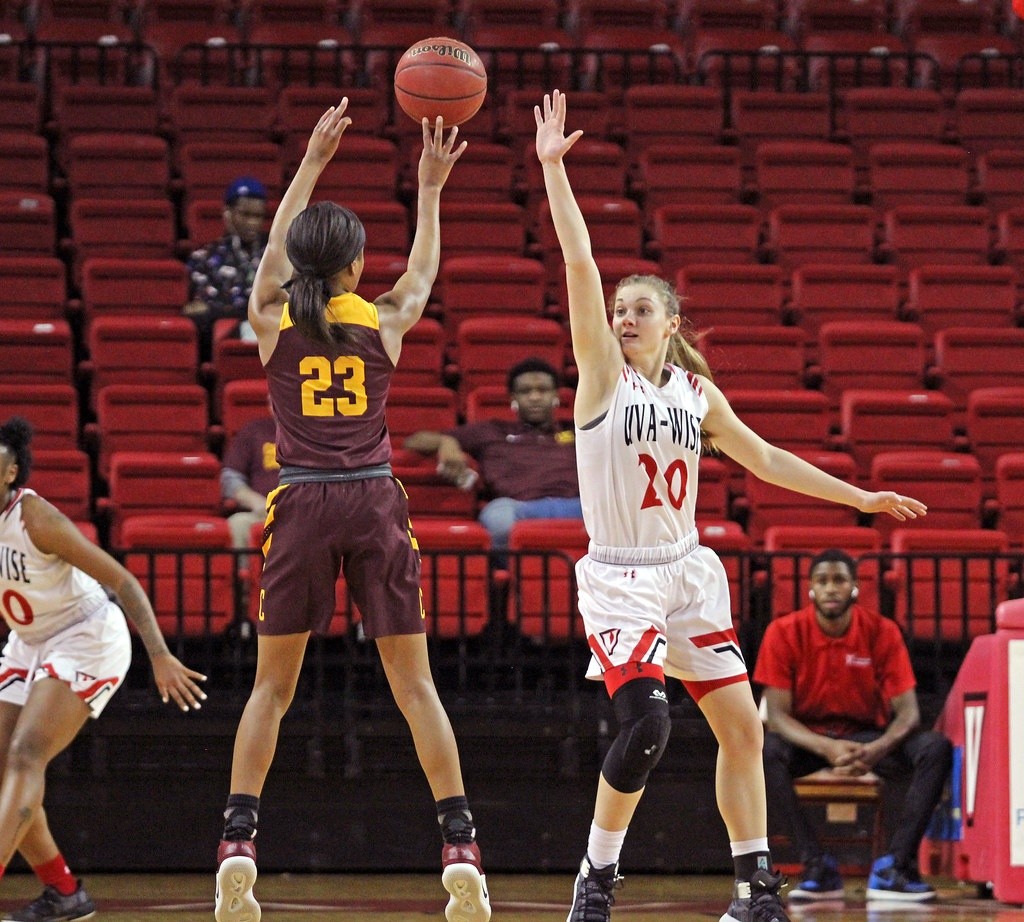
[1,0,1022,645]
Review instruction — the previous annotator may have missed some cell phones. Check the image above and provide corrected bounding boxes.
[436,463,479,491]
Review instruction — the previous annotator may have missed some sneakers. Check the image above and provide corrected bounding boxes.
[566,857,619,922]
[441,840,493,922]
[215,839,262,922]
[866,856,938,902]
[790,849,845,900]
[719,869,792,922]
[1,880,95,922]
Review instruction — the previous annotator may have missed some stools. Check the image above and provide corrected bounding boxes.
[767,766,890,879]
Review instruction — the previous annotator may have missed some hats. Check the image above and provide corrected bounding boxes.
[225,176,266,202]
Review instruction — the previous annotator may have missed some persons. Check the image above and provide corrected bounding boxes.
[404,355,585,573]
[533,85,930,921]
[211,91,495,922]
[752,546,955,901]
[1,418,210,921]
[189,176,273,343]
[221,394,286,571]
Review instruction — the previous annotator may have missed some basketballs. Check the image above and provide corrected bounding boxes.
[393,37,487,129]
